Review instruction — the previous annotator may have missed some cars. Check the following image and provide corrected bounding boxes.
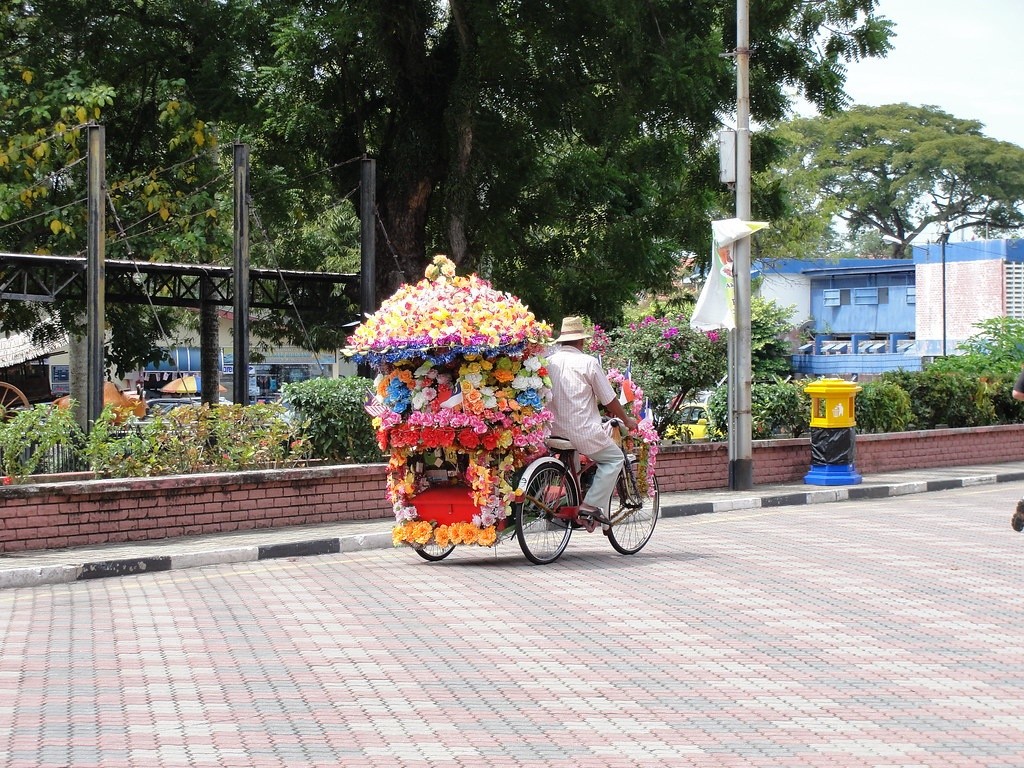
[126,387,305,435]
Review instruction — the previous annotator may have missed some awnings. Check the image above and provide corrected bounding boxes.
[144,346,225,374]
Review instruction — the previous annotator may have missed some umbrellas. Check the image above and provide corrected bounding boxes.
[160,374,228,396]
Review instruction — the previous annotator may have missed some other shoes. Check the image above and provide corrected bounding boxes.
[1011,499,1024,532]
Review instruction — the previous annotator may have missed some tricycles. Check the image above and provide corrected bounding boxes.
[341,254,660,563]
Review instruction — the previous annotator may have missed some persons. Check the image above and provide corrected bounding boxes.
[541,315,640,525]
[1012,366,1024,532]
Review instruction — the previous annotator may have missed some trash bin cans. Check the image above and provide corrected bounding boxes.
[804,379,862,486]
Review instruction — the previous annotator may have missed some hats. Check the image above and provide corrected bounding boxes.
[556,315,593,342]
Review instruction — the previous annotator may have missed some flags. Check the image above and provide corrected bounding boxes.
[620,358,634,405]
[638,398,653,429]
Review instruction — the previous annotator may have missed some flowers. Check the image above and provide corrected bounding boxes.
[344,252,718,547]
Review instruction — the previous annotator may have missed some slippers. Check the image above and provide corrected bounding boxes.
[578,502,613,526]
